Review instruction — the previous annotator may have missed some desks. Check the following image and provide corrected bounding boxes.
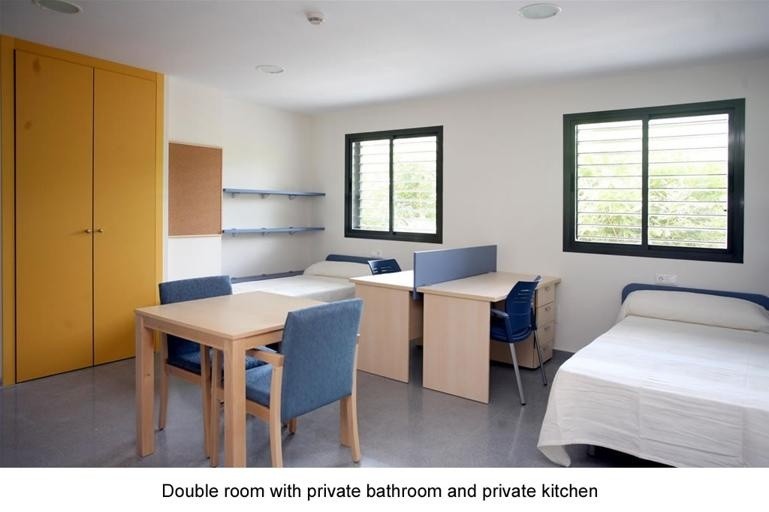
[133,290,331,467]
[349,266,563,404]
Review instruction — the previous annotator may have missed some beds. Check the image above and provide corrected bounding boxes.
[230,254,382,302]
[537,282,768,466]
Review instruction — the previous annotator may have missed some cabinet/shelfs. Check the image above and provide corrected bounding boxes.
[0,32,167,388]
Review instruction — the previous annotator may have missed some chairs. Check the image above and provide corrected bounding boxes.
[368,257,402,274]
[158,277,364,467]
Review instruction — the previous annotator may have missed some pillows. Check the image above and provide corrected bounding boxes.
[626,289,765,332]
[303,260,372,279]
